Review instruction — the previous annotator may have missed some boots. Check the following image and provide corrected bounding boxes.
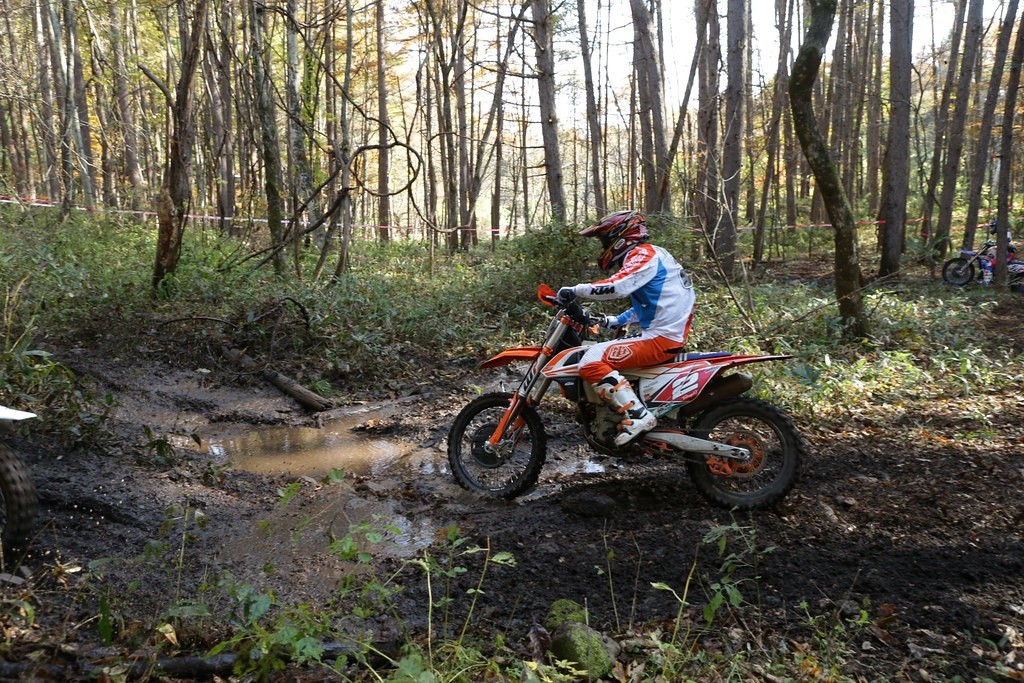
[591,370,657,446]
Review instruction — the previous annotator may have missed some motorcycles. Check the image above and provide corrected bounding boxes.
[0,407,38,566]
[447,283,807,513]
[942,239,1024,293]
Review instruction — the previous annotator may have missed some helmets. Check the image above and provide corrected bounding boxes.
[990,221,997,235]
[578,209,650,277]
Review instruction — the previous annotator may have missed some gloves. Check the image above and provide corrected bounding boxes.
[598,316,619,329]
[986,242,995,248]
[557,286,578,305]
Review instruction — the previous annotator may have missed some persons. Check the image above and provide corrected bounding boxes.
[984,221,1016,283]
[556,211,696,449]
